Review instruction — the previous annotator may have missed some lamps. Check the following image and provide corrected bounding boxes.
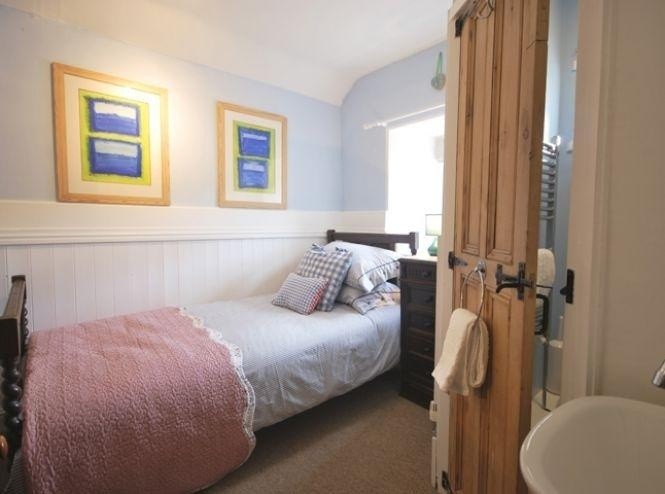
[424,214,443,257]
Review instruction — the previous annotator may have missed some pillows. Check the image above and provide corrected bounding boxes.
[271,238,402,316]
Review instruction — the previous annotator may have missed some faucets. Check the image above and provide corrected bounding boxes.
[652,363,664,389]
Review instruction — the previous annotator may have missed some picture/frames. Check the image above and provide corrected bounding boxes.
[216,100,287,210]
[50,61,172,207]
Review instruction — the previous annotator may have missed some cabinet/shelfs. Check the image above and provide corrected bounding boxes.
[398,254,437,411]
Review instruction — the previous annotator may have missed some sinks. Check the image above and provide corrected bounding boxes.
[519,396,664,494]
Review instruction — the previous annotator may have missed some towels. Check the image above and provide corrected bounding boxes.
[532,248,556,331]
[431,308,490,397]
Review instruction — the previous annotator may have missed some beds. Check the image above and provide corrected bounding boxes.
[0,229,418,494]
